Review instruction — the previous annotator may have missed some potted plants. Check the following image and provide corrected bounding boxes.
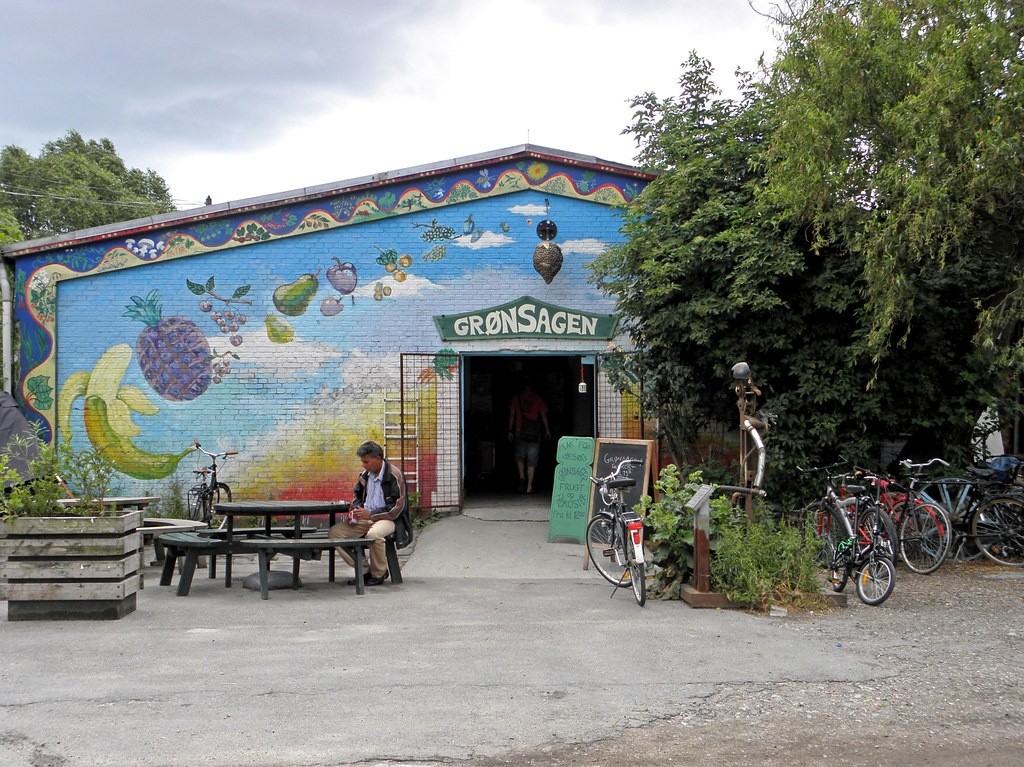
[0,417,143,622]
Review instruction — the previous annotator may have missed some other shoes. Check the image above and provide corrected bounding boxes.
[527,487,533,494]
[519,475,527,484]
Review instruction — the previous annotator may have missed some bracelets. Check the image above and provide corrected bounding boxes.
[508,431,511,432]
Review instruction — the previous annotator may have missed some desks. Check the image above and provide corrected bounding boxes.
[213,500,351,589]
[56,496,160,589]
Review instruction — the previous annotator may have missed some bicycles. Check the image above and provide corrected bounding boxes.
[797,452,1024,607]
[586,459,647,608]
[187,438,239,532]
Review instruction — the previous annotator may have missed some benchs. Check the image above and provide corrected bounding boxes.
[160,527,403,596]
[137,517,208,573]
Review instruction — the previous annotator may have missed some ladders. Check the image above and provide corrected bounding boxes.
[383,388,420,501]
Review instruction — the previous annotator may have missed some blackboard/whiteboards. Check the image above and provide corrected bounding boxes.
[587,437,659,547]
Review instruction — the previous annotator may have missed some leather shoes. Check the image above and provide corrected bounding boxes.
[365,569,389,586]
[347,572,372,585]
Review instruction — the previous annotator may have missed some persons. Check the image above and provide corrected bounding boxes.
[329,441,408,585]
[508,378,551,494]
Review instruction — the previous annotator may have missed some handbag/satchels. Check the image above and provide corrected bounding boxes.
[519,418,539,443]
